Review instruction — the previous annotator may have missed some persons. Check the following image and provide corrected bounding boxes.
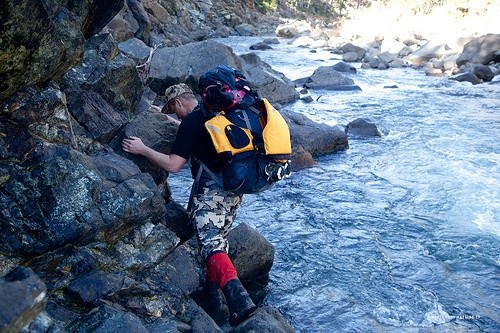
[122,82,256,326]
